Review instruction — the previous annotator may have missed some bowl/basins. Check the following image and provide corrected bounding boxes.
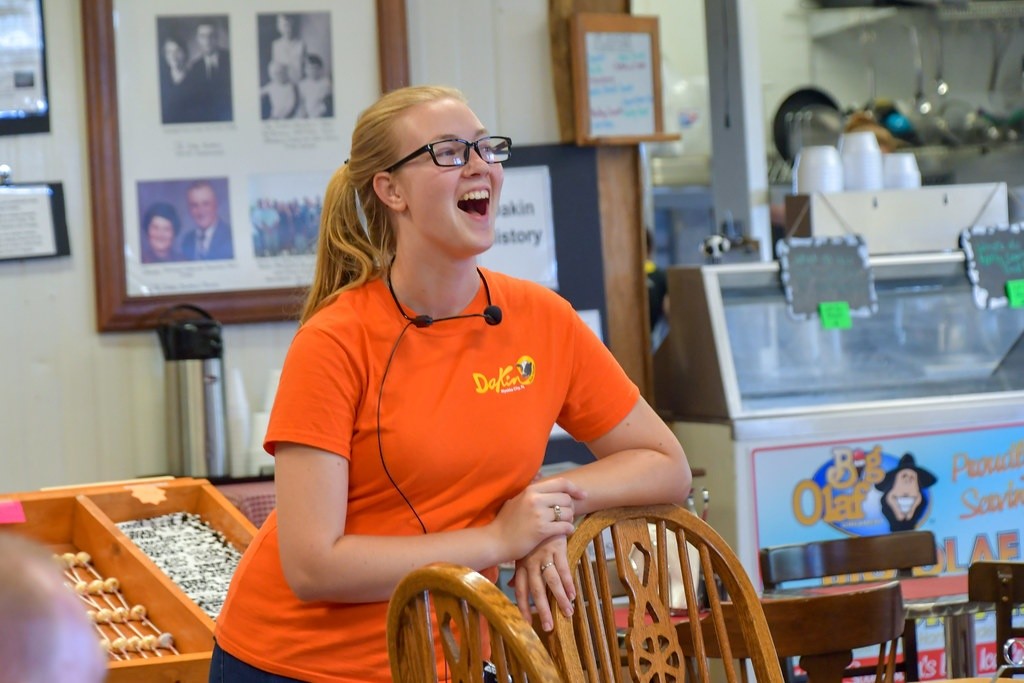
[791,131,923,194]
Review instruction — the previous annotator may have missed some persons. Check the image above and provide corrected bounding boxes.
[206,85,693,683]
[261,14,331,119]
[646,229,671,351]
[142,182,232,263]
[161,20,230,123]
[252,195,323,256]
[0,533,107,682]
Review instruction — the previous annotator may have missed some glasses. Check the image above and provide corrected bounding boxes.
[385,136,513,173]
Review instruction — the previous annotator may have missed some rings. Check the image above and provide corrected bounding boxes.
[541,563,552,571]
[554,505,561,521]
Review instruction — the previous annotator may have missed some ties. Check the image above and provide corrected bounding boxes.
[199,232,205,252]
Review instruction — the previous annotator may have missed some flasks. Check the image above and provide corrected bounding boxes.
[157,305,231,481]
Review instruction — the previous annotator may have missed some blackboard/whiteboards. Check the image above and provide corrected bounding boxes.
[568,13,680,145]
[777,235,877,323]
[960,222,1024,310]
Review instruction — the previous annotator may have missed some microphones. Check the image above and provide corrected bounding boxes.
[413,305,502,329]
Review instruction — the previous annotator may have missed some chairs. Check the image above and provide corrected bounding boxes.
[383,493,1024,683]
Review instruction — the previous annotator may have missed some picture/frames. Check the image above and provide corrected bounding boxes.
[77,1,412,336]
